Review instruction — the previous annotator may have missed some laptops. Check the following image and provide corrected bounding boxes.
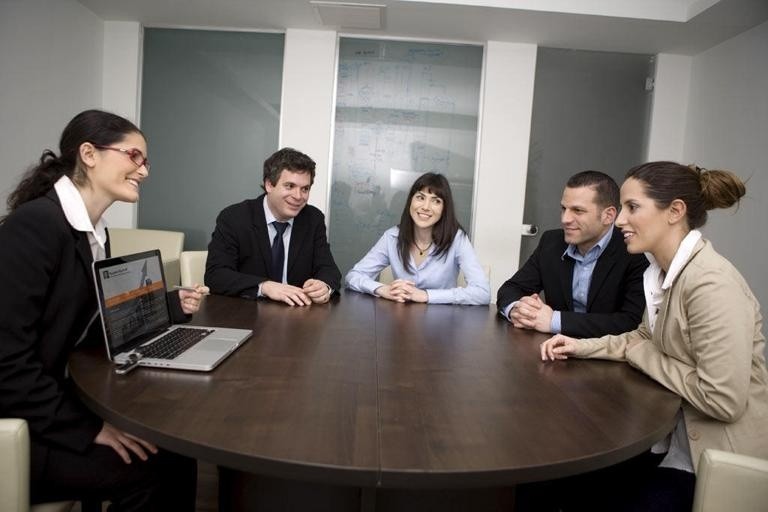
[91,249,253,372]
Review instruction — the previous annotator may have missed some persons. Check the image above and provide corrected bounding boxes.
[538,161,768,511]
[202,147,344,307]
[345,173,491,307]
[497,171,652,339]
[1,109,210,511]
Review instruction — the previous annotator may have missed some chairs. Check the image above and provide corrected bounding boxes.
[0,417,76,512]
[378,262,491,289]
[691,447,768,512]
[104,226,186,293]
[179,250,209,290]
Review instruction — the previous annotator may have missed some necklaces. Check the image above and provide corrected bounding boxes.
[414,241,433,255]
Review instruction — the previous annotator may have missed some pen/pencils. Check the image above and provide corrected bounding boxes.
[173,285,211,296]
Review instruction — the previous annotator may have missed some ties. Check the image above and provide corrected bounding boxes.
[272,221,288,284]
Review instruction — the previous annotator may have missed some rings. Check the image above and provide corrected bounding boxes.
[322,294,327,300]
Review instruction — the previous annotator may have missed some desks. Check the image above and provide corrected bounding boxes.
[67,294,684,511]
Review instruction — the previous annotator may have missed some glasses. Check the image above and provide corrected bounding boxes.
[95,146,151,172]
